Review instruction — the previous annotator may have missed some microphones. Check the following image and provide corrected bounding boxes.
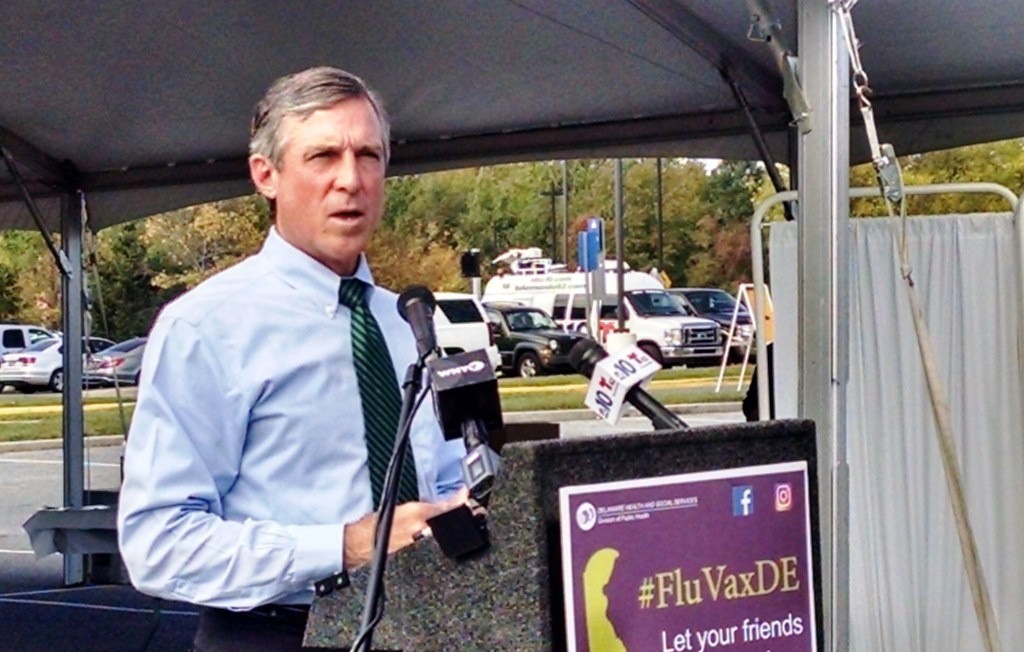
[396,284,506,508]
[568,339,690,431]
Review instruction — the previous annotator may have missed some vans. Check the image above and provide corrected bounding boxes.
[485,261,723,372]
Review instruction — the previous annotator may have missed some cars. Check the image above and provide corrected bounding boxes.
[1,323,149,392]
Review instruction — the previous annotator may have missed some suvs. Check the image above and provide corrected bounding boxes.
[480,302,602,378]
[431,290,503,382]
[670,287,758,365]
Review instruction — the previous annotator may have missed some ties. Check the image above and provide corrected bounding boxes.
[339,278,420,513]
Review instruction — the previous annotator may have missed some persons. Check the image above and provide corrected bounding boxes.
[115,65,487,652]
